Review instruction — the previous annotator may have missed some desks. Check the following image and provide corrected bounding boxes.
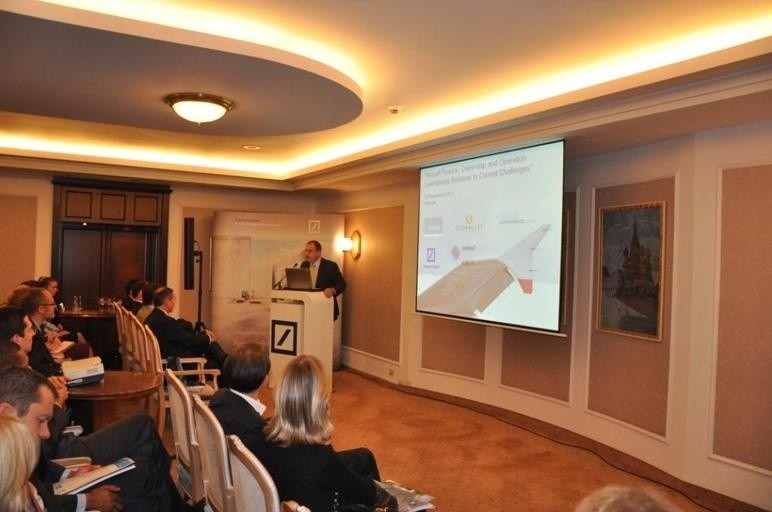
[51,370,163,436]
[54,301,117,361]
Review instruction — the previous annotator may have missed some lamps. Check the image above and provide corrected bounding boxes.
[164,91,236,127]
[336,230,361,260]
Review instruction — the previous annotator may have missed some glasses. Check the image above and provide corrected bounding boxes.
[40,302,57,308]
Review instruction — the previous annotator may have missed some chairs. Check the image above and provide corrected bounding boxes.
[114,300,146,371]
[165,369,207,503]
[192,392,235,511]
[226,434,310,512]
[145,324,221,436]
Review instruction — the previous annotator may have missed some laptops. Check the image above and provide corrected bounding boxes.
[285,268,324,290]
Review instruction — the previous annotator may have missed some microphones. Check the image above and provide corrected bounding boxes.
[273,262,298,289]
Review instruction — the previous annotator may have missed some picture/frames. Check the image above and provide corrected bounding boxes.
[595,200,667,342]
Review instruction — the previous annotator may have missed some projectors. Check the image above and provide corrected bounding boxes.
[60,356,105,387]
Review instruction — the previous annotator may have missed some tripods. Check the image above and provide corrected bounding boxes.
[192,251,207,335]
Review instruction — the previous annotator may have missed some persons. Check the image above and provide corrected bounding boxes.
[573,484,682,512]
[0,275,228,512]
[300,240,347,322]
[208,342,380,512]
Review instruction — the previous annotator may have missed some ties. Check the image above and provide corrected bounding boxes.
[40,324,46,337]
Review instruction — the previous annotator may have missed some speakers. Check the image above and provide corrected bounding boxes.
[184,218,194,289]
